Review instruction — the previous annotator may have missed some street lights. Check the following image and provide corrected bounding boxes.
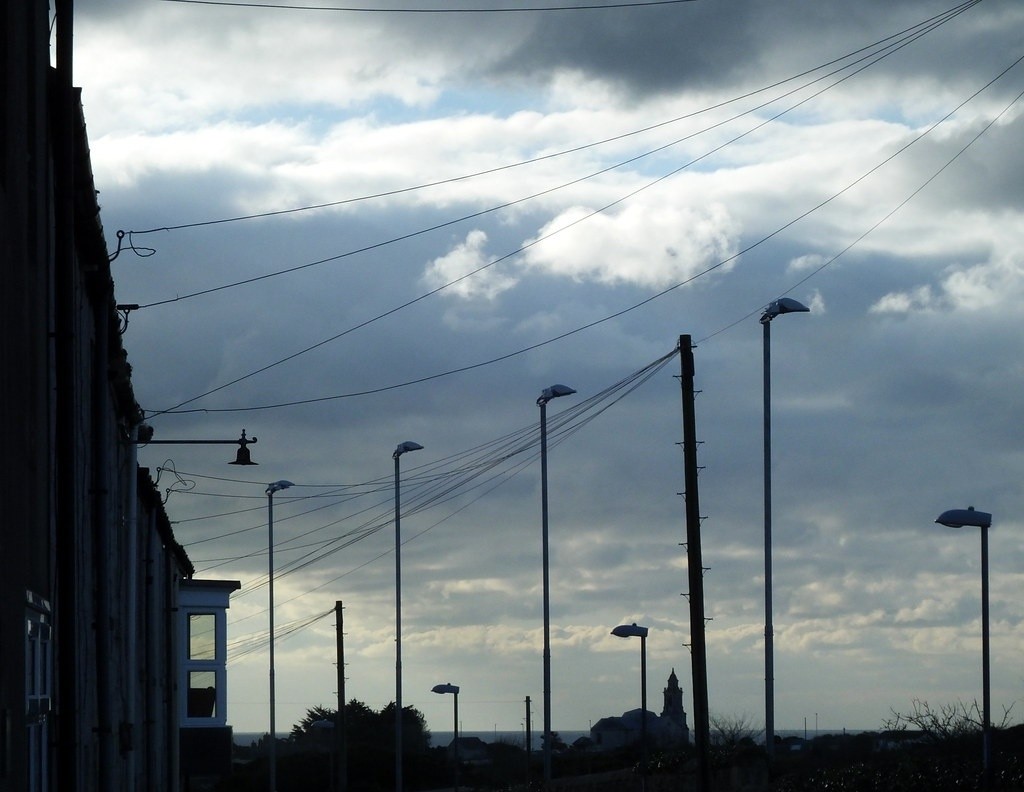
[935,506,993,774]
[760,297,812,791]
[537,384,579,791]
[393,441,424,791]
[611,623,649,792]
[431,682,459,792]
[266,479,295,791]
